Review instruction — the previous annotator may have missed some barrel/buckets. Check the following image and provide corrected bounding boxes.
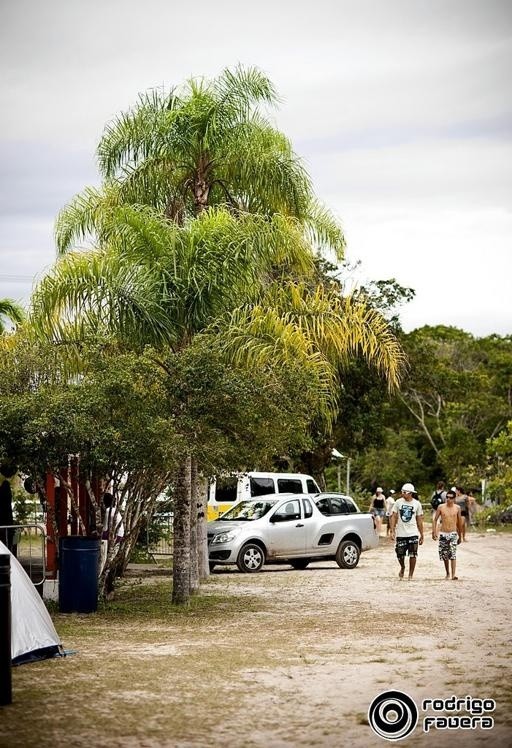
[59,536,99,614]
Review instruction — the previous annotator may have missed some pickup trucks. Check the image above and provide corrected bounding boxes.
[207,494,379,572]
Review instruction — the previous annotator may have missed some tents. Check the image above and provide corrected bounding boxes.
[1,539,78,668]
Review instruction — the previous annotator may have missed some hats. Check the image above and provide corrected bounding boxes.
[376,487,384,492]
[401,483,418,494]
[390,490,396,494]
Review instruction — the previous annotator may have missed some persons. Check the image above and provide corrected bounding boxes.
[102,493,124,543]
[385,490,396,536]
[431,479,480,543]
[432,490,462,580]
[390,483,424,579]
[369,487,386,533]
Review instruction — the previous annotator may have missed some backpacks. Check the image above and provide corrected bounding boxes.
[431,490,444,509]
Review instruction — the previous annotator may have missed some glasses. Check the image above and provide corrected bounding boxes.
[402,505,413,510]
[402,491,411,494]
[446,496,455,499]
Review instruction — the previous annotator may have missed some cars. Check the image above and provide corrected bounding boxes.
[316,493,361,513]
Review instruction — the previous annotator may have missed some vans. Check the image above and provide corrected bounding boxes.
[207,472,323,523]
